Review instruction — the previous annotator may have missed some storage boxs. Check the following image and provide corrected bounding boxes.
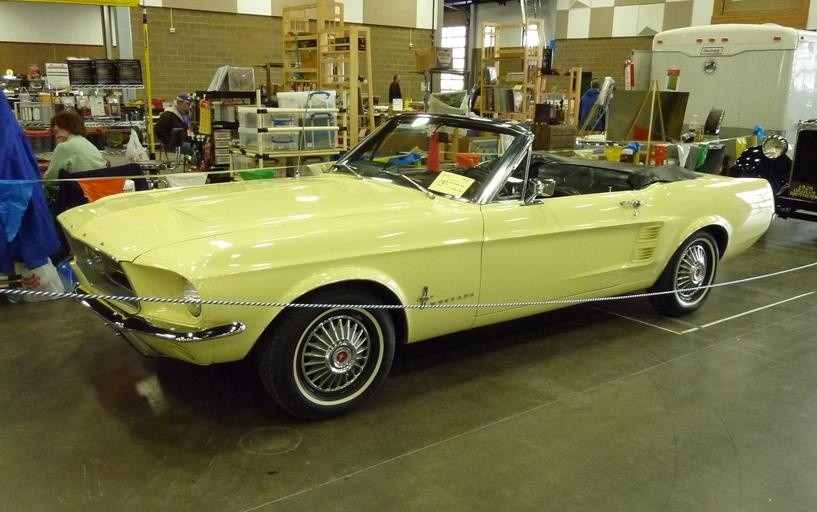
[237,88,338,157]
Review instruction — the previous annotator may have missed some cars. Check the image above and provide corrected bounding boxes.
[727,115,816,224]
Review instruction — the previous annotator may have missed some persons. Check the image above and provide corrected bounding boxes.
[39,109,110,199]
[19,65,44,97]
[580,78,605,131]
[154,94,195,156]
[389,74,402,103]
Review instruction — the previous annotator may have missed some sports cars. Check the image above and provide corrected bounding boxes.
[55,111,779,424]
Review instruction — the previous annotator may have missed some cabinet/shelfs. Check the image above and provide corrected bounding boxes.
[280,0,376,152]
[479,19,545,125]
